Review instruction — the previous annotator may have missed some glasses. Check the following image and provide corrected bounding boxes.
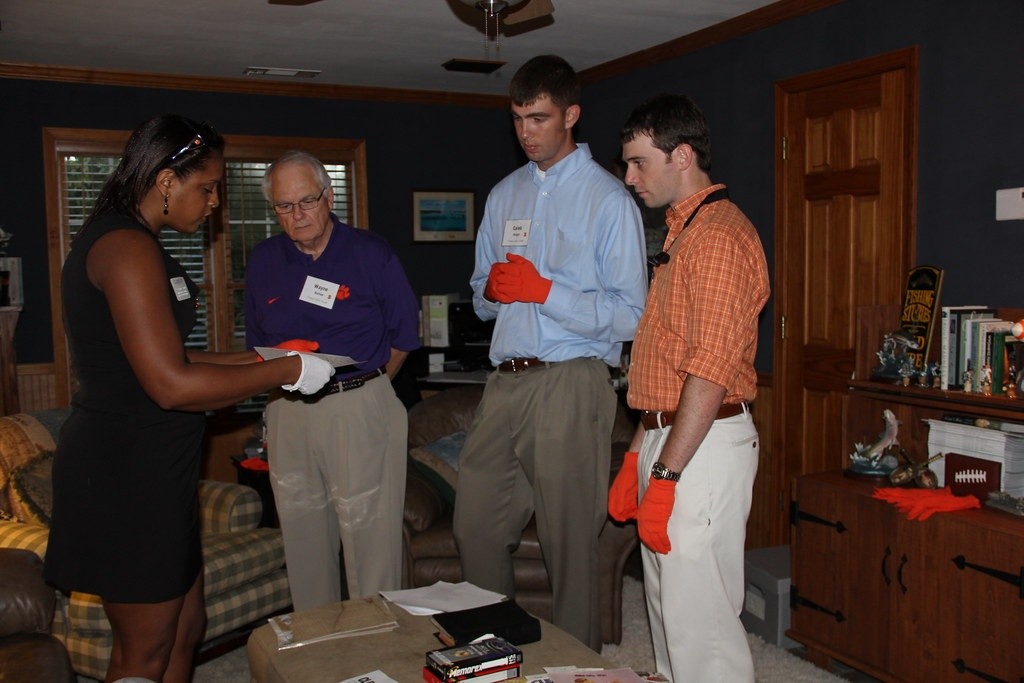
[647,227,684,267]
[146,119,218,183]
[272,187,325,214]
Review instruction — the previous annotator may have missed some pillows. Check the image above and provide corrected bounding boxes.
[409,428,468,511]
[10,447,53,529]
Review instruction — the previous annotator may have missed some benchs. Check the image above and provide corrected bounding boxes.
[247,597,617,683]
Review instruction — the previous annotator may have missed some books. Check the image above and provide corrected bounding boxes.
[423,638,523,683]
[431,598,542,648]
[926,412,1024,499]
[941,306,1024,395]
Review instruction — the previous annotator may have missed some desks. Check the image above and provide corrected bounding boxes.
[0,306,23,417]
[417,371,488,392]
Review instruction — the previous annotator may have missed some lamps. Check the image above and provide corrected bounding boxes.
[458,0,522,52]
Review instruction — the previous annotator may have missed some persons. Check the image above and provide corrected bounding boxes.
[243,150,424,612]
[39,110,337,683]
[451,55,649,657]
[608,94,772,683]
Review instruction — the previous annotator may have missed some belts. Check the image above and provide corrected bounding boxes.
[497,357,538,373]
[277,365,387,404]
[640,401,746,431]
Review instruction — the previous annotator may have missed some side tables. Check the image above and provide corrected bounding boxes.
[232,460,281,528]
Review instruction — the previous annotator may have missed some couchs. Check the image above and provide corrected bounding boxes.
[400,382,640,646]
[0,407,292,683]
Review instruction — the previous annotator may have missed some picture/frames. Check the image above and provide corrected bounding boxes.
[409,186,479,246]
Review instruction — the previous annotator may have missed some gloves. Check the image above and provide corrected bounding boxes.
[871,482,981,522]
[257,339,319,364]
[485,261,508,303]
[495,253,552,305]
[281,350,337,396]
[608,452,639,523]
[637,474,677,555]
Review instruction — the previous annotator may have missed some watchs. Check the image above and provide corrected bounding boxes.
[651,462,680,481]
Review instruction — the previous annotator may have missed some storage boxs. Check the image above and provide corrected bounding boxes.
[422,663,521,683]
[425,637,524,682]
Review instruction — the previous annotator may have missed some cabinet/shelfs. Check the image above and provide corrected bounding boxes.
[783,474,1024,683]
